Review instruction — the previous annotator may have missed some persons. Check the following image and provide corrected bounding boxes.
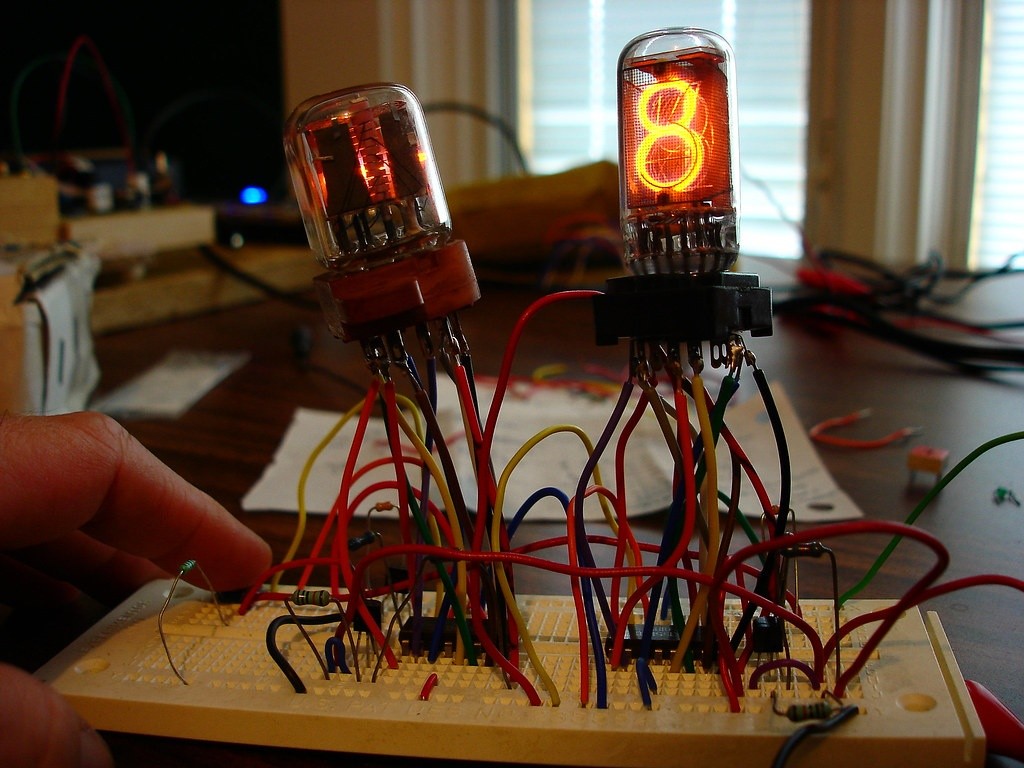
[0,412,273,768]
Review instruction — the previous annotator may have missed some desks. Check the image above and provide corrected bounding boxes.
[0,242,1024,767]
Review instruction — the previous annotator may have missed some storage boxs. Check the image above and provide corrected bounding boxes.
[1,246,103,418]
